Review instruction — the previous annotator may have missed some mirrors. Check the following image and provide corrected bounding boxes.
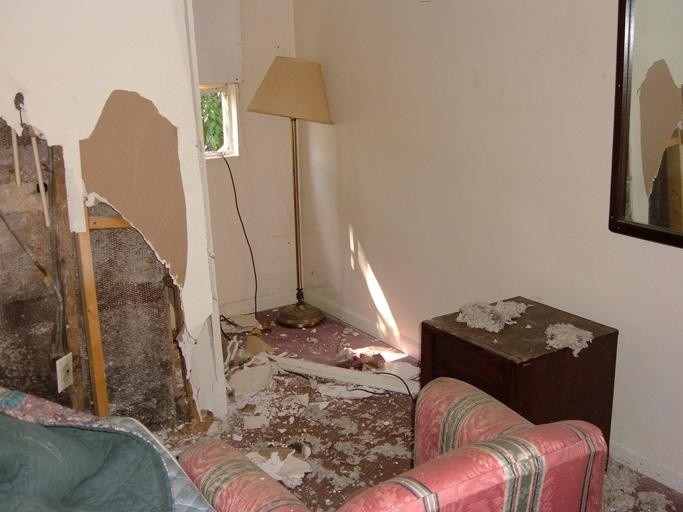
[606,2,682,248]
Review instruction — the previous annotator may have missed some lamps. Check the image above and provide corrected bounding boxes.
[245,54,335,331]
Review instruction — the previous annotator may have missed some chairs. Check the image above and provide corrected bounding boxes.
[174,373,608,512]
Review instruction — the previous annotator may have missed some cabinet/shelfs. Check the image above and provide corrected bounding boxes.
[418,295,619,445]
